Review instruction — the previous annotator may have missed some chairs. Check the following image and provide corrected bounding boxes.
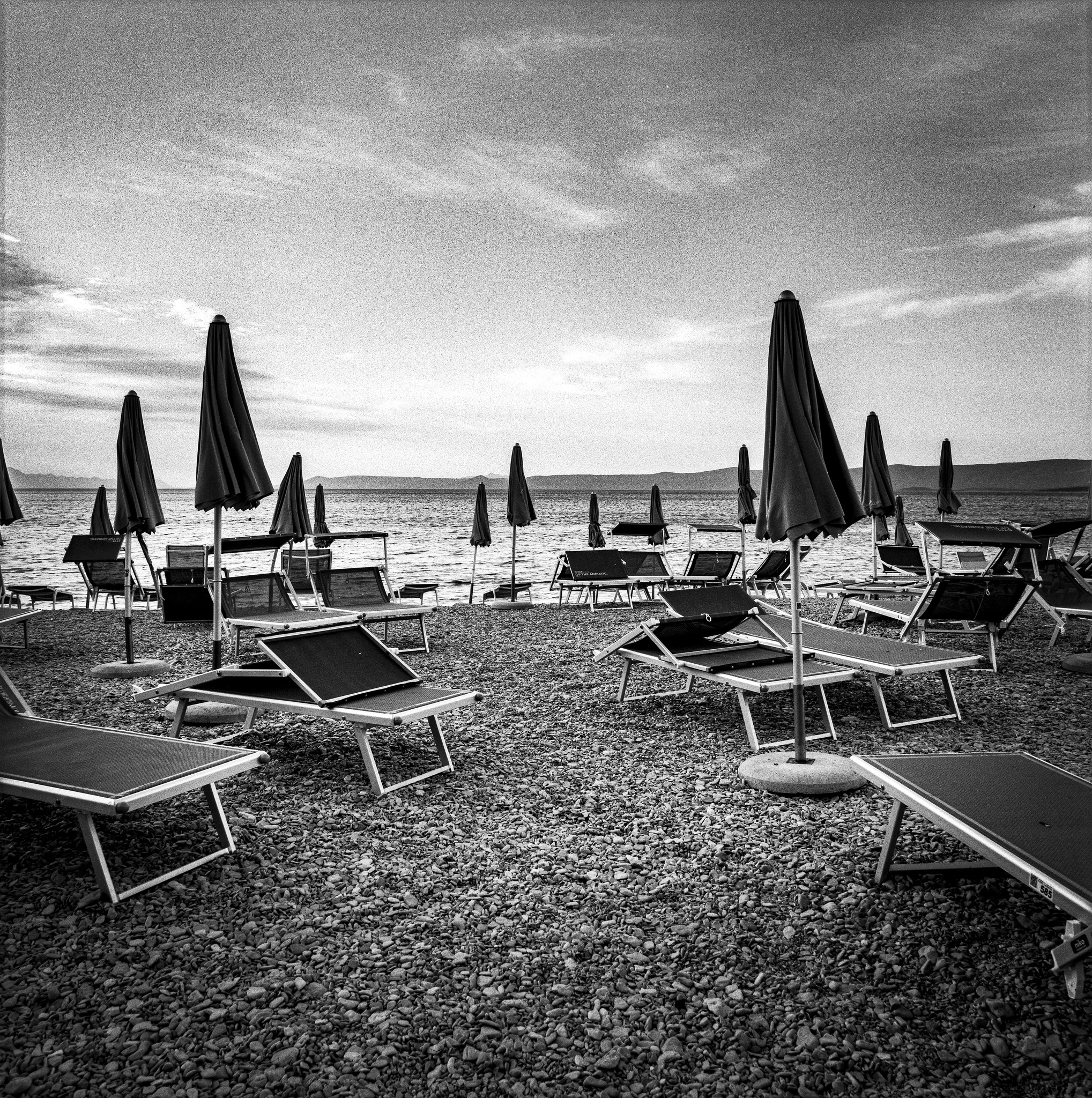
[549,515,1092,753]
[1,531,483,906]
[483,583,533,604]
[850,750,1092,998]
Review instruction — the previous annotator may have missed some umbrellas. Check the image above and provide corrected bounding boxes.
[937,437,963,568]
[468,482,491,604]
[760,289,864,763]
[88,486,118,539]
[645,484,670,600]
[0,439,24,600]
[118,390,168,664]
[268,453,311,594]
[862,410,895,602]
[508,442,538,603]
[192,317,272,668]
[315,480,334,577]
[894,493,913,550]
[736,444,757,593]
[589,491,607,603]
